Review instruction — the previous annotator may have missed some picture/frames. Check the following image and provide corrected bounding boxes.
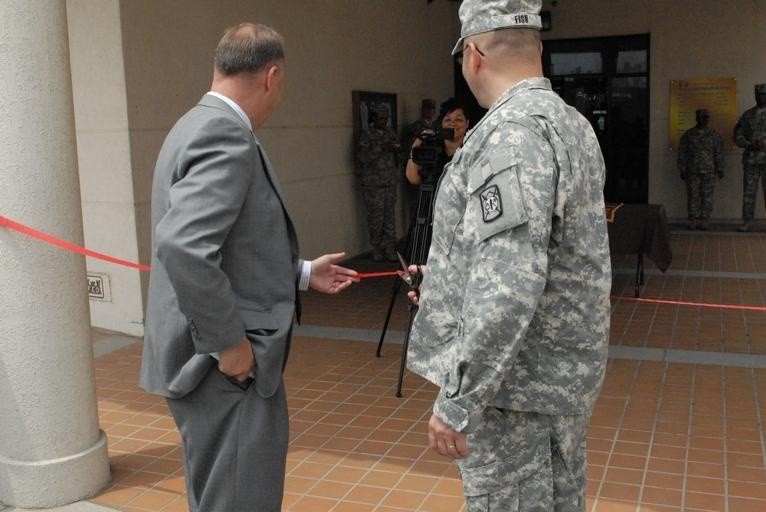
[351,89,399,144]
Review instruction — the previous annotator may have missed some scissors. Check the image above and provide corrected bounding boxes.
[396,252,422,308]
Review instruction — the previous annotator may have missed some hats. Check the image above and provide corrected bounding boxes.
[422,97,438,110]
[371,104,391,119]
[754,83,766,94]
[451,0,543,55]
[695,108,711,118]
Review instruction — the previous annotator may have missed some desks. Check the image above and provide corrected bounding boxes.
[602,200,669,301]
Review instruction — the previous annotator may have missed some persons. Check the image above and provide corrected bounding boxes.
[732,81,766,233]
[354,97,473,263]
[406,0,616,511]
[133,23,359,512]
[676,108,726,231]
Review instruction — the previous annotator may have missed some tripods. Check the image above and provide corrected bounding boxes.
[376,183,437,398]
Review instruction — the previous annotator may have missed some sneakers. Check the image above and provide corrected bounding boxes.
[735,220,755,233]
[689,220,698,231]
[383,241,400,262]
[700,219,711,230]
[371,243,385,261]
